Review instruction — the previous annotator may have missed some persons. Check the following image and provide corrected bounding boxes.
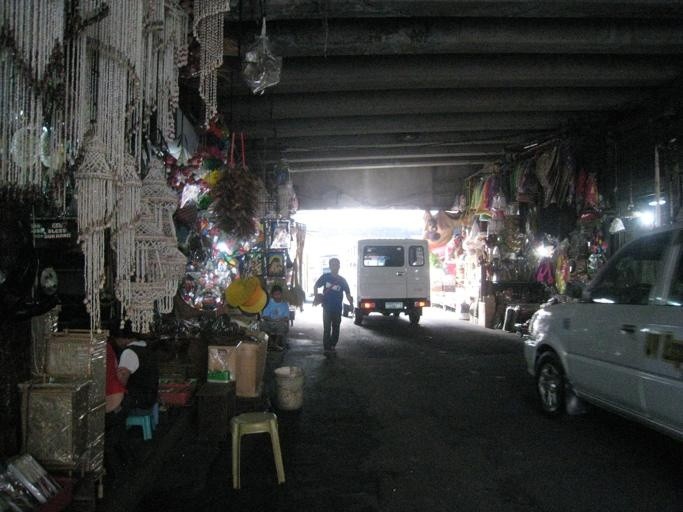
[105,343,132,428]
[314,258,353,356]
[110,325,158,410]
[261,285,290,352]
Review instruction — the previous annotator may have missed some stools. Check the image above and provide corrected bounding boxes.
[126,401,159,441]
[229,411,286,488]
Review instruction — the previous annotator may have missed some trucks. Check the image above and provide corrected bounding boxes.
[341,236,432,326]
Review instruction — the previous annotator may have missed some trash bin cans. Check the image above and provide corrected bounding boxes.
[274,365,305,412]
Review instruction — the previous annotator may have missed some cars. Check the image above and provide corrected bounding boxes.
[524,221,682,444]
